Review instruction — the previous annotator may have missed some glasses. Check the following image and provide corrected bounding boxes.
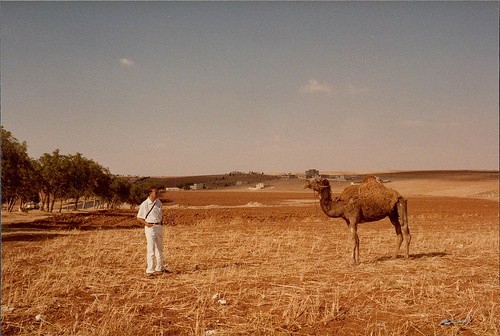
[150,191,158,194]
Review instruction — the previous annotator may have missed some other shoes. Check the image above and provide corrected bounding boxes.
[145,269,173,274]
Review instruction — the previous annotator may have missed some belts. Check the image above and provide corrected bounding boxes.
[148,222,161,225]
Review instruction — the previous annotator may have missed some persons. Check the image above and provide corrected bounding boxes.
[137,185,171,277]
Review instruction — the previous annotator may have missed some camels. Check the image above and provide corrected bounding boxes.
[302,172,412,266]
